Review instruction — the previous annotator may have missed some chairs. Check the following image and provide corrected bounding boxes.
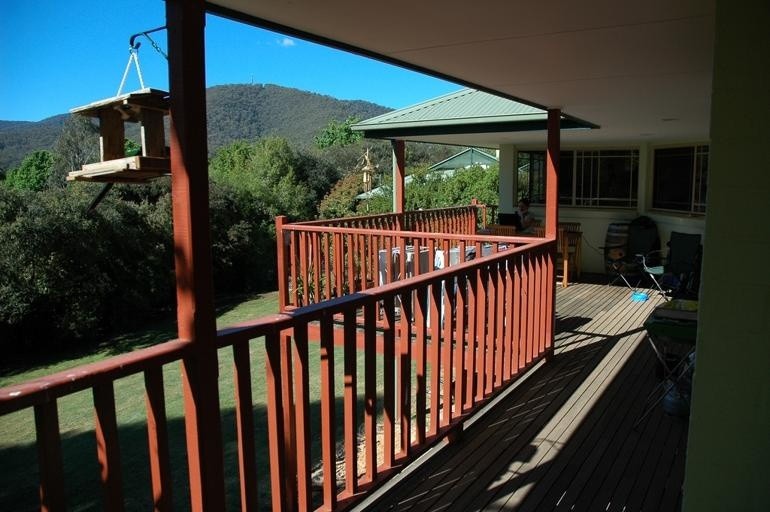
[608,215,703,429]
[476,218,584,287]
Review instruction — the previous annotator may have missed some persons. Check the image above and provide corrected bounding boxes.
[513,199,537,235]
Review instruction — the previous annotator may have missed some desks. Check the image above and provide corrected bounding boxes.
[378,246,478,326]
